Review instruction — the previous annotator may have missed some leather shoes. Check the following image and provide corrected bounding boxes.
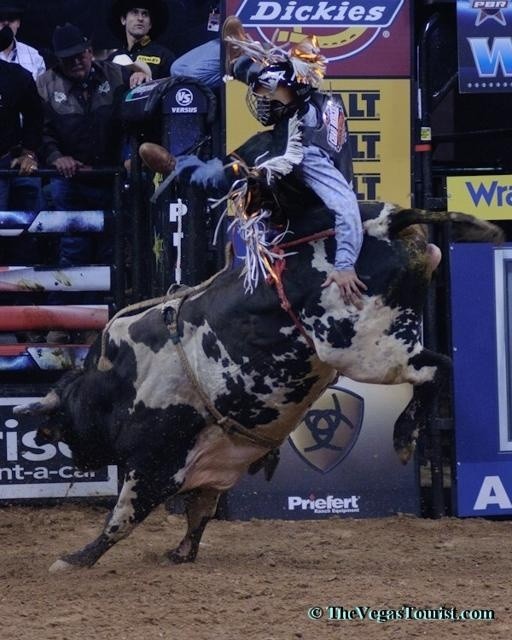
[139,142,176,172]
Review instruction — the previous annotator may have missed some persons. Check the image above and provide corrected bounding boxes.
[138,14,367,311]
[0,3,220,385]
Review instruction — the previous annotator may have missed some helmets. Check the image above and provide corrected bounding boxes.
[245,57,314,126]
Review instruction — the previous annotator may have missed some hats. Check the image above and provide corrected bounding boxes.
[54,23,87,59]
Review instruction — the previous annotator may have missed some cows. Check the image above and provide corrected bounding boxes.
[12,201,504,575]
